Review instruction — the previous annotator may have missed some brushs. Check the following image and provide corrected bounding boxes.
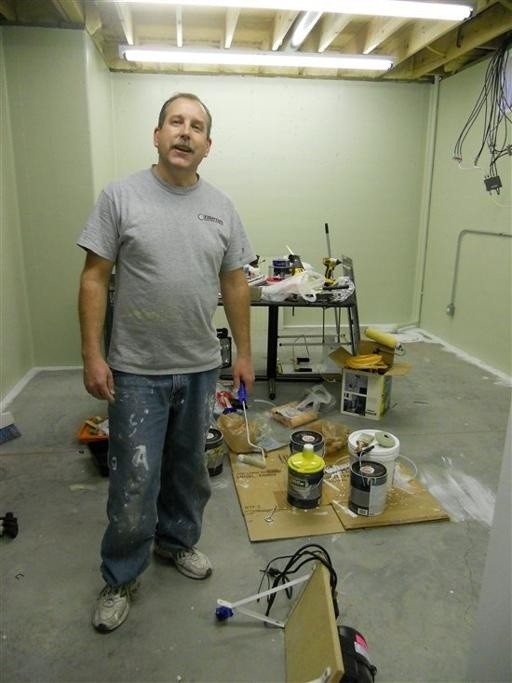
[355,432,376,453]
[236,383,268,469]
[360,326,407,356]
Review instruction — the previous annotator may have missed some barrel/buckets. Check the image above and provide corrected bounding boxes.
[349,461,388,516]
[289,430,325,457]
[347,430,400,490]
[206,429,225,476]
[287,452,325,509]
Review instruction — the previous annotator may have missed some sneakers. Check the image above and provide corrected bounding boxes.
[153,542,212,579]
[93,576,140,631]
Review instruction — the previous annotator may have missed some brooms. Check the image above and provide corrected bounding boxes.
[0,411,22,444]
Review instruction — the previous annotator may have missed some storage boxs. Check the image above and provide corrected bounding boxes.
[328,340,411,420]
[77,415,109,477]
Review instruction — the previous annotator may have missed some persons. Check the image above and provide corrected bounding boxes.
[78,95,255,633]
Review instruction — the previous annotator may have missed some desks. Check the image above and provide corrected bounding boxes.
[217,296,357,399]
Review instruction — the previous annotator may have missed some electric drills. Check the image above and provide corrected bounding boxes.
[321,257,343,286]
